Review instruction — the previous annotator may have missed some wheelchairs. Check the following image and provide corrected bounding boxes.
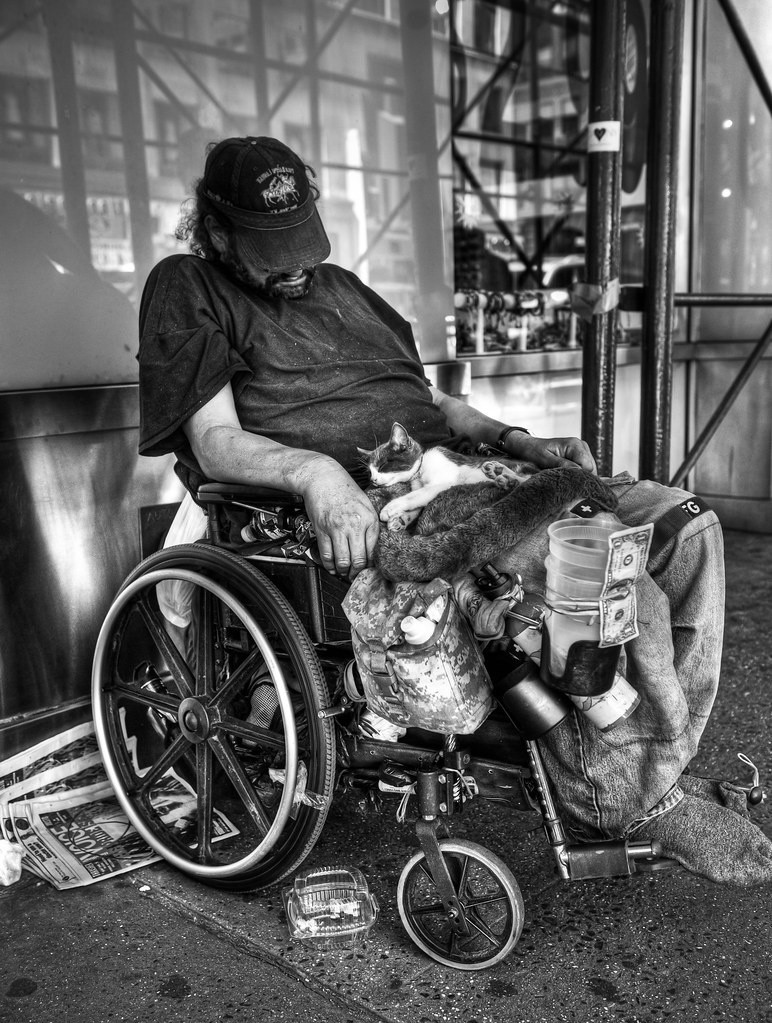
[86,483,677,970]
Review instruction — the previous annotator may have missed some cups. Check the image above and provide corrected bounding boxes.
[543,519,634,675]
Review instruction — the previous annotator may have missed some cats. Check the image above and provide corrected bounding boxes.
[355,421,538,532]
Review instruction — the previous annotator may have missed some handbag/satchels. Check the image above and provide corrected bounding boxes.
[340,566,496,735]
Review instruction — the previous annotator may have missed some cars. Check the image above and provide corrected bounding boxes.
[504,253,587,291]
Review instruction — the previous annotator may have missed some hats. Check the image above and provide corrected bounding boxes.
[198,137,331,273]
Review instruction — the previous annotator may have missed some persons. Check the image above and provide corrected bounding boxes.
[139,136,772,885]
